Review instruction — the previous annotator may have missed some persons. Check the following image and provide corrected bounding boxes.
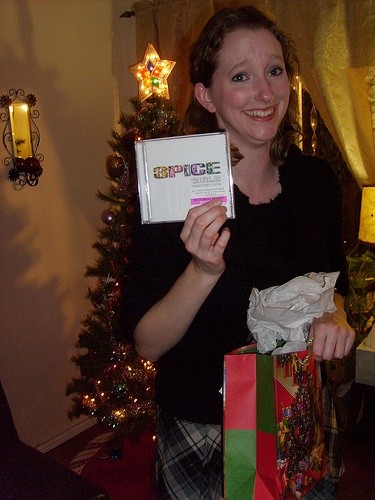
[120,10,355,500]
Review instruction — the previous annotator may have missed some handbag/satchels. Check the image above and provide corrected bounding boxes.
[221,339,346,500]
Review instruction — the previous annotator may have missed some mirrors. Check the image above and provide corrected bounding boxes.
[0,88,44,186]
